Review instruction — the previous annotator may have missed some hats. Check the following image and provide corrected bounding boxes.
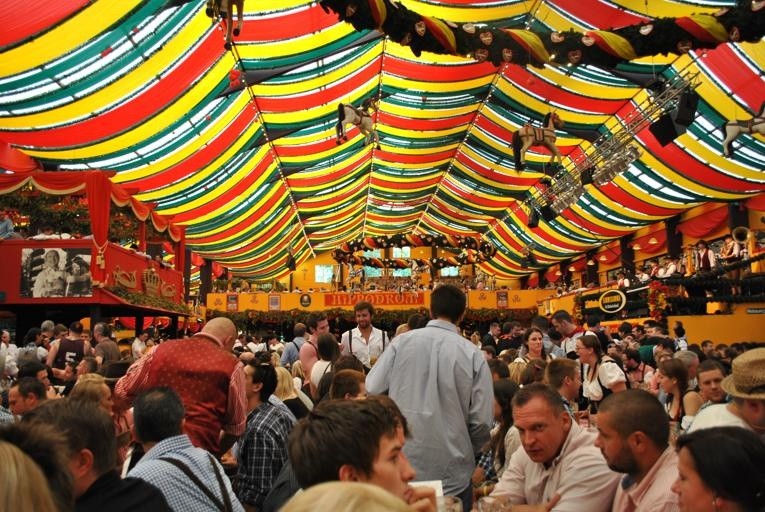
[719,347,765,401]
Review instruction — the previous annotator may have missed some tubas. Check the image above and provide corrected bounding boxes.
[733,226,751,249]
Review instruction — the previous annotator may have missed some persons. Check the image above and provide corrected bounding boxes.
[0,231,764,512]
[25,217,76,243]
[0,214,15,241]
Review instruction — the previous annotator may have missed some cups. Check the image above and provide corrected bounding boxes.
[479,495,512,512]
[668,422,682,442]
[428,494,462,512]
[579,414,598,432]
